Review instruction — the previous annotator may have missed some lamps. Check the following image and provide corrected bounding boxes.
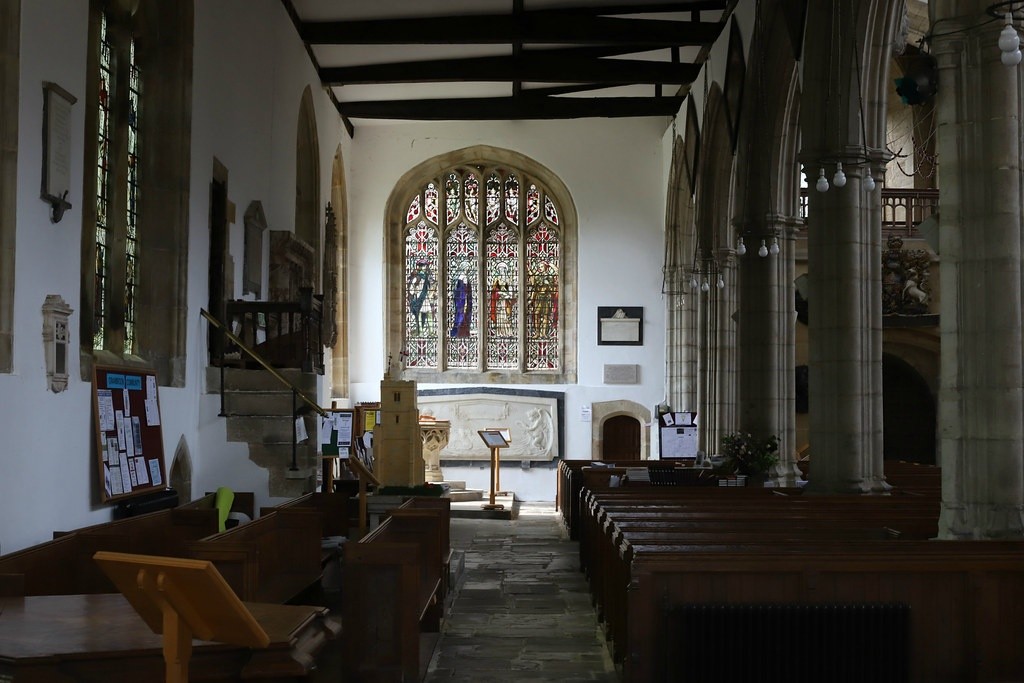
[815,0,875,193]
[687,55,725,292]
[985,0,1024,66]
[732,0,781,258]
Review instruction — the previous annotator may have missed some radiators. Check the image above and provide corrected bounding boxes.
[655,584,914,683]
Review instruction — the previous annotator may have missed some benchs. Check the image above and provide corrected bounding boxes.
[0,490,452,683]
[556,456,1024,683]
